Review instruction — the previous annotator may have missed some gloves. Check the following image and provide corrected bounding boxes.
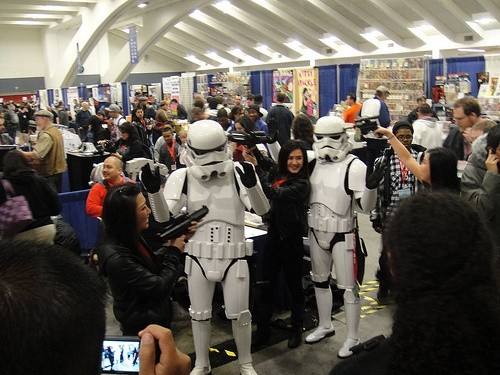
[140,163,161,194]
[235,160,257,188]
[365,162,388,190]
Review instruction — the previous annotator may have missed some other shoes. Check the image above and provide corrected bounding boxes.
[190,366,212,375]
[337,337,361,358]
[305,324,335,344]
[240,363,259,375]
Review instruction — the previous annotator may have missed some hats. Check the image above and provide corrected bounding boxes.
[376,85,390,94]
[33,109,54,117]
[104,103,121,113]
[247,104,264,117]
[392,119,414,135]
[138,97,148,101]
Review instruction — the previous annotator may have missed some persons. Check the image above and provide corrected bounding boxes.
[265,115,383,358]
[97,184,197,365]
[0,90,316,192]
[103,344,139,370]
[241,142,311,348]
[360,85,391,166]
[3,149,64,223]
[460,126,500,245]
[343,93,363,130]
[375,125,459,193]
[406,96,440,123]
[1,239,192,375]
[366,119,429,303]
[329,192,500,374]
[85,156,135,217]
[471,120,498,143]
[139,118,270,375]
[452,97,488,161]
[410,103,447,150]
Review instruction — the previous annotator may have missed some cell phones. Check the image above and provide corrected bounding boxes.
[101,334,161,375]
[485,144,496,154]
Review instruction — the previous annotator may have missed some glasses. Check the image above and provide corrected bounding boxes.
[396,135,413,141]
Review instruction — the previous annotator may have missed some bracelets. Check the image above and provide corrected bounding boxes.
[388,134,395,140]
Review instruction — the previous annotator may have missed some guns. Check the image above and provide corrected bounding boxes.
[345,115,383,138]
[156,204,209,243]
[229,131,278,158]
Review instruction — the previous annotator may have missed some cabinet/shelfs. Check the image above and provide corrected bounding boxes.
[39,57,500,125]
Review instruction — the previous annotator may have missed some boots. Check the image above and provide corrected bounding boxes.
[253,303,274,347]
[288,303,305,348]
